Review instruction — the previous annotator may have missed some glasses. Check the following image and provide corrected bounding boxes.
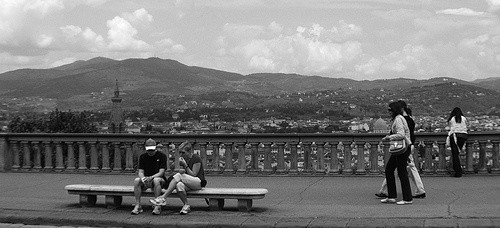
[388,108,392,111]
[147,149,155,152]
[179,151,186,154]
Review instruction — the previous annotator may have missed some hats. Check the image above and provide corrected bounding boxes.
[145,139,156,150]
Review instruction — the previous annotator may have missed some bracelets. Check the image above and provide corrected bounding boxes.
[185,165,189,169]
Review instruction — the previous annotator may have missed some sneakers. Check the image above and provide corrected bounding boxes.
[180,204,190,214]
[149,196,167,206]
[375,193,389,198]
[151,206,161,215]
[397,199,412,205]
[381,198,396,203]
[131,205,144,215]
[413,193,426,198]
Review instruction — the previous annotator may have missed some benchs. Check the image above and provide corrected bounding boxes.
[64,184,268,211]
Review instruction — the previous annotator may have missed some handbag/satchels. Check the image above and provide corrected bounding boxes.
[389,139,407,155]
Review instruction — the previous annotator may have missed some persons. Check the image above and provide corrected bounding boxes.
[445,107,470,178]
[375,100,427,198]
[380,102,414,205]
[131,138,168,214]
[149,142,207,215]
